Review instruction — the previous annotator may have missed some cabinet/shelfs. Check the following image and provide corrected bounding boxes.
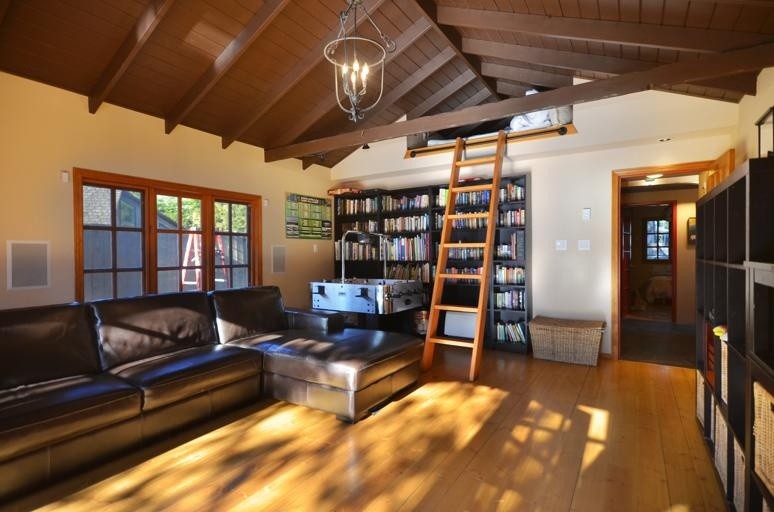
[695,154,774,506]
[333,173,532,355]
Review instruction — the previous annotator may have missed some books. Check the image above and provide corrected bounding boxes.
[335,183,527,347]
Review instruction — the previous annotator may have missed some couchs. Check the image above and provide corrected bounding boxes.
[0,286,426,512]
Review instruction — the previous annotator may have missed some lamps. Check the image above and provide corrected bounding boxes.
[322,1,396,125]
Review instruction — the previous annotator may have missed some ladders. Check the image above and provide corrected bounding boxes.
[177,227,229,291]
[421,130,507,383]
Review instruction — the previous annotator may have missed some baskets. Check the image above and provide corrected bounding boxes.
[752,379,774,499]
